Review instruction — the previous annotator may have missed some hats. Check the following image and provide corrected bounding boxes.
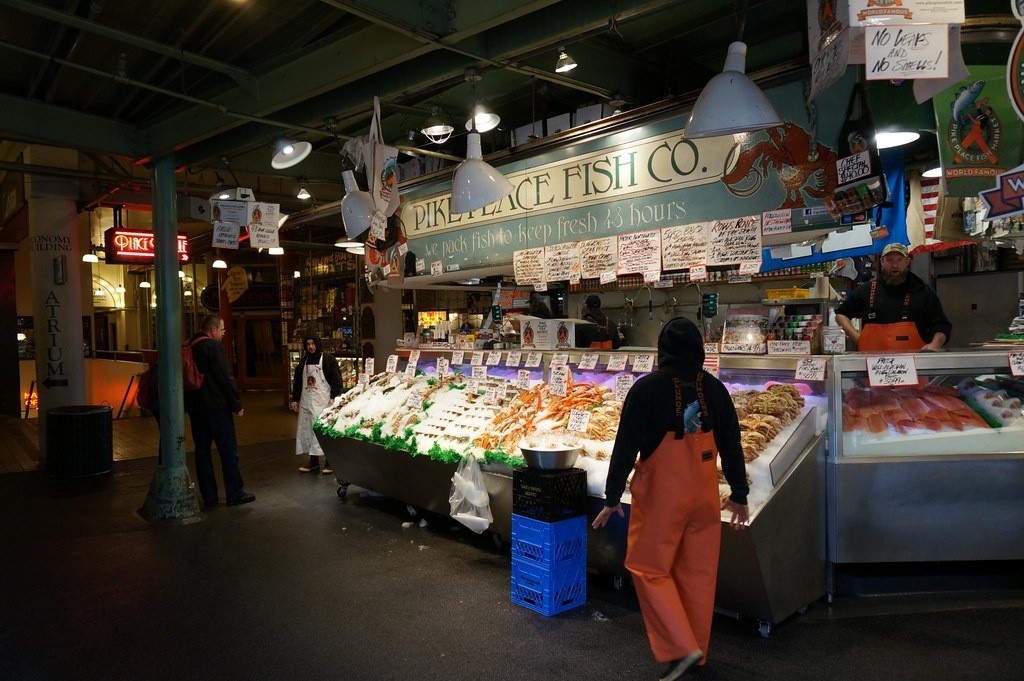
[881,242,908,257]
[586,295,601,309]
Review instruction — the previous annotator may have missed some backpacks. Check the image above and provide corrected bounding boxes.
[182,336,209,390]
[136,369,157,409]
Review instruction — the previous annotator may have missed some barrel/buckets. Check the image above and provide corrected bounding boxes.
[45,404,113,493]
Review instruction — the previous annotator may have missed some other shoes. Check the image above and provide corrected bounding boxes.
[204,496,218,506]
[132,507,150,525]
[298,464,320,472]
[323,467,334,474]
[227,494,255,506]
[659,650,704,681]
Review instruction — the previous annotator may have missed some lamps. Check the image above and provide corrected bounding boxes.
[680,0,785,139]
[209,170,237,201]
[420,67,515,213]
[555,47,577,73]
[393,129,419,158]
[324,117,376,255]
[297,186,311,199]
[271,136,312,169]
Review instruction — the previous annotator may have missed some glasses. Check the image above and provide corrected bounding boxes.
[214,327,226,333]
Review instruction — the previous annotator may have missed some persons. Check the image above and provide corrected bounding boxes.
[836,243,952,355]
[290,335,343,474]
[592,317,750,681]
[183,313,256,509]
[148,358,162,470]
[524,297,552,320]
[572,294,621,350]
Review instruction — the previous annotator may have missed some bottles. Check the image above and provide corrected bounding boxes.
[770,314,823,341]
[835,178,884,214]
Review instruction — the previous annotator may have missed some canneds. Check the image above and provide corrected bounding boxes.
[768,315,823,341]
[753,265,801,277]
[570,275,644,291]
[660,269,747,283]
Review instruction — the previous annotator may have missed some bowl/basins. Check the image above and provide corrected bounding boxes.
[517,444,583,468]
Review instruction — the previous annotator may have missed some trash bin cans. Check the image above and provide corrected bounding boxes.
[46,404,115,478]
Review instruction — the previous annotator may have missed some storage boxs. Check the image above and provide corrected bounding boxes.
[511,467,587,616]
[419,328,494,349]
[509,315,591,350]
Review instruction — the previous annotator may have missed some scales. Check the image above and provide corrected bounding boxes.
[700,291,720,342]
[492,304,502,325]
